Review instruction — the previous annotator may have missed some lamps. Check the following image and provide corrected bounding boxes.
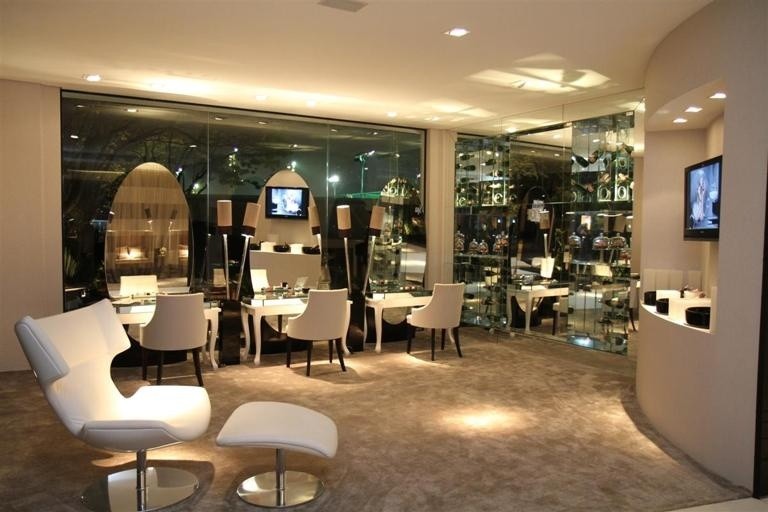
[235,202,261,301]
[108,211,115,225]
[307,207,322,255]
[337,205,352,297]
[361,206,385,295]
[216,200,233,300]
[142,208,155,231]
[161,208,178,248]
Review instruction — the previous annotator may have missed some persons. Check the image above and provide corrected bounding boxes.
[689,177,708,222]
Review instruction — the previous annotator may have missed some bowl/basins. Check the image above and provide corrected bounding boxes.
[643,290,657,305]
[249,242,261,251]
[684,306,711,329]
[273,245,290,252]
[654,297,670,315]
[303,246,322,255]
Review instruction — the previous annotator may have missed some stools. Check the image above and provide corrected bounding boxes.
[215,401,339,509]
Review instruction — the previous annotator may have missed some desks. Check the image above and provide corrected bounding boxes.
[506,284,569,331]
[241,298,354,366]
[116,307,222,369]
[363,292,455,354]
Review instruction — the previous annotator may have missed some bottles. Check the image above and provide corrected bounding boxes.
[453,230,508,258]
[455,140,509,197]
[567,232,581,250]
[591,232,627,249]
[572,139,634,167]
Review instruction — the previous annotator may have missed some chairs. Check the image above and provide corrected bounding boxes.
[286,288,349,377]
[14,298,212,512]
[139,293,209,386]
[406,281,465,361]
[250,268,269,294]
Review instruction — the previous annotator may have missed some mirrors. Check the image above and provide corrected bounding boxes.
[105,162,194,344]
[249,170,322,335]
[506,121,569,343]
[367,176,426,326]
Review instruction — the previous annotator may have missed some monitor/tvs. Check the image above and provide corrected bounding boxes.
[265,186,309,219]
[683,155,722,240]
